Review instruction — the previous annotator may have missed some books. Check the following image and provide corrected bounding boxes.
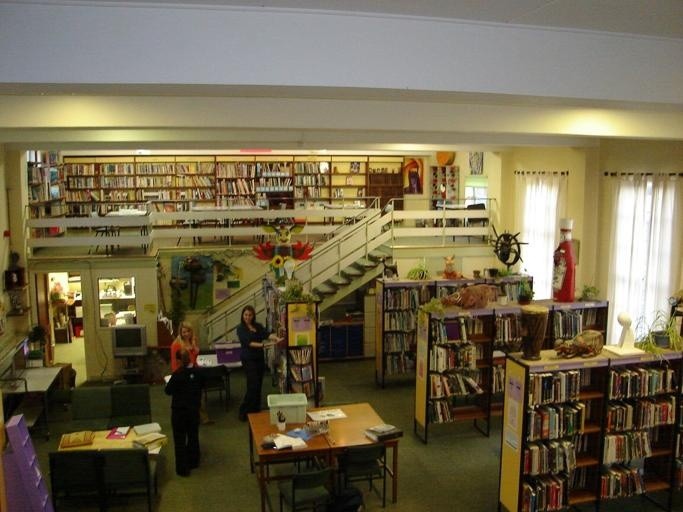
[364,424,403,442]
[332,163,363,198]
[65,164,325,214]
[385,285,431,377]
[133,421,162,435]
[521,370,588,512]
[59,428,94,447]
[131,431,167,450]
[496,282,522,301]
[601,365,676,498]
[29,164,68,236]
[438,287,449,299]
[104,426,131,440]
[427,314,524,424]
[553,309,597,339]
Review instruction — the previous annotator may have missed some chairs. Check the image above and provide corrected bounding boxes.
[49,448,157,512]
[278,442,386,512]
[85,200,154,255]
[107,384,152,430]
[321,197,379,241]
[201,365,230,412]
[467,204,485,244]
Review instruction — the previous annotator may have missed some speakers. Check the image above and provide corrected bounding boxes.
[5,266,25,290]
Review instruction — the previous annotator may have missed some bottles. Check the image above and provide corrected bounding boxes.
[550,218,574,302]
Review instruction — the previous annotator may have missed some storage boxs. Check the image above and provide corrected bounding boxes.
[214,342,241,364]
[267,393,308,425]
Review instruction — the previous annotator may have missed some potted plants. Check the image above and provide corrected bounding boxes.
[577,284,600,303]
[516,276,535,304]
[407,261,432,281]
[632,309,682,364]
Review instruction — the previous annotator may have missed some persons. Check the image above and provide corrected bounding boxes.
[169,320,215,426]
[165,347,200,476]
[235,303,280,422]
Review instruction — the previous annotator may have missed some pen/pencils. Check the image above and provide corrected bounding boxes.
[277,410,285,422]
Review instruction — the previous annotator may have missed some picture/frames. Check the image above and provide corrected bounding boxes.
[168,254,216,314]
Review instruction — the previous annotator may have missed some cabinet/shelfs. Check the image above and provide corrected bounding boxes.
[215,156,255,226]
[486,277,534,306]
[331,155,367,226]
[98,155,134,228]
[435,279,486,305]
[263,274,319,407]
[554,305,607,346]
[135,156,175,227]
[294,154,331,226]
[610,351,682,512]
[62,155,98,228]
[378,278,436,388]
[499,360,610,511]
[413,307,493,444]
[255,155,294,224]
[492,305,555,417]
[52,300,70,343]
[4,283,28,317]
[318,323,364,360]
[175,155,215,226]
[30,161,64,238]
[367,155,404,224]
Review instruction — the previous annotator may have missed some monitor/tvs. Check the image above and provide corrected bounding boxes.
[111,324,147,357]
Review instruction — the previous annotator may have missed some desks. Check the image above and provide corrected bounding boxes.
[3,367,68,441]
[174,202,286,246]
[58,429,162,503]
[436,204,467,242]
[306,402,400,503]
[247,411,331,512]
